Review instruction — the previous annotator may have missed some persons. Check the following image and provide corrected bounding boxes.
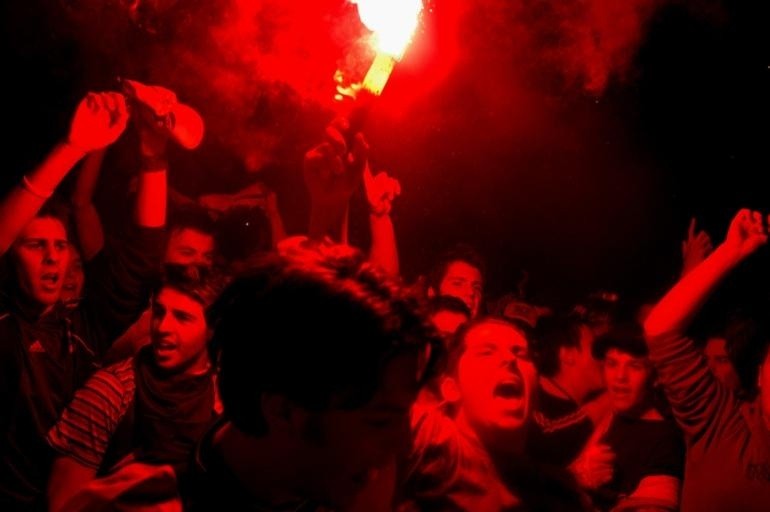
[1,81,770,511]
